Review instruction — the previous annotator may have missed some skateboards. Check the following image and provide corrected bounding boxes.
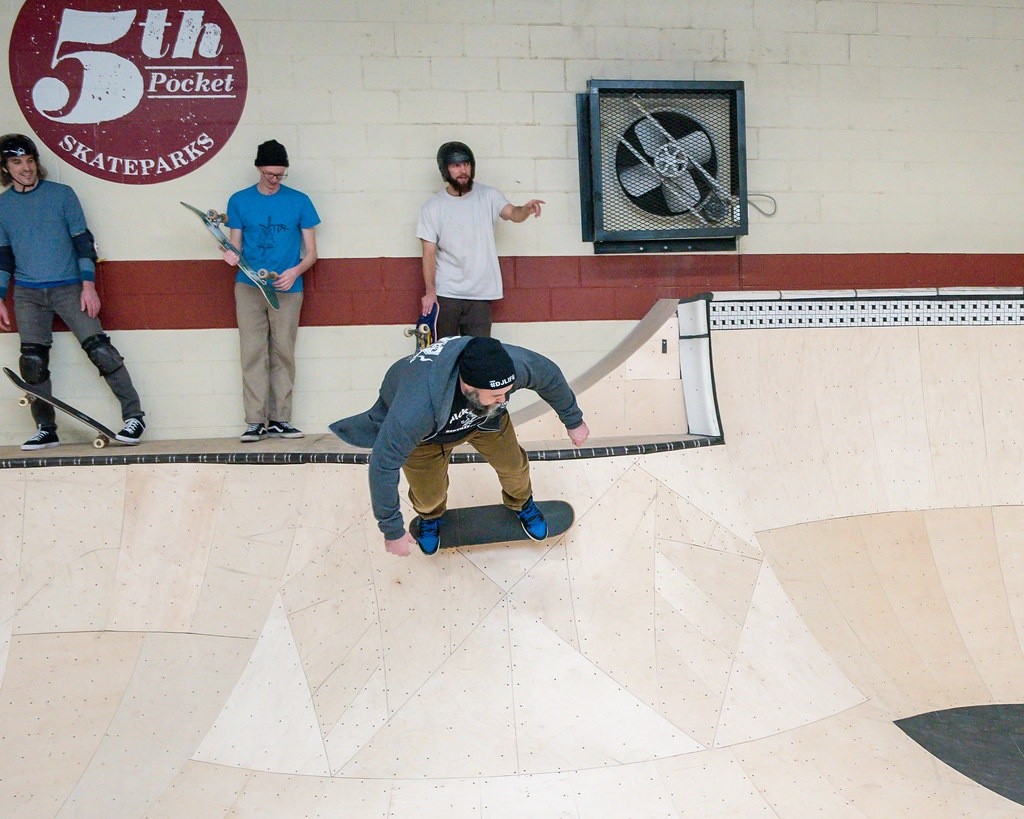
[409,499,575,550]
[3,367,139,449]
[404,300,440,353]
[180,201,280,311]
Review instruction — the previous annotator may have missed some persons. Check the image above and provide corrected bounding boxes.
[0,133,147,451]
[218,140,321,442]
[329,336,590,557]
[416,142,544,338]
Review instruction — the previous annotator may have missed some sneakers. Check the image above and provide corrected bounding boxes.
[267,421,305,438]
[115,417,145,444]
[515,495,549,542]
[21,423,59,450]
[240,422,268,441]
[416,516,441,555]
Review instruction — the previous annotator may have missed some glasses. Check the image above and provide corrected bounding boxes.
[261,166,289,181]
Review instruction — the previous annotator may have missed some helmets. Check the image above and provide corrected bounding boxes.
[437,142,475,183]
[0,134,38,162]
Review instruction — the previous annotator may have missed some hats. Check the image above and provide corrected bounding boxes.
[459,337,517,389]
[255,139,289,167]
